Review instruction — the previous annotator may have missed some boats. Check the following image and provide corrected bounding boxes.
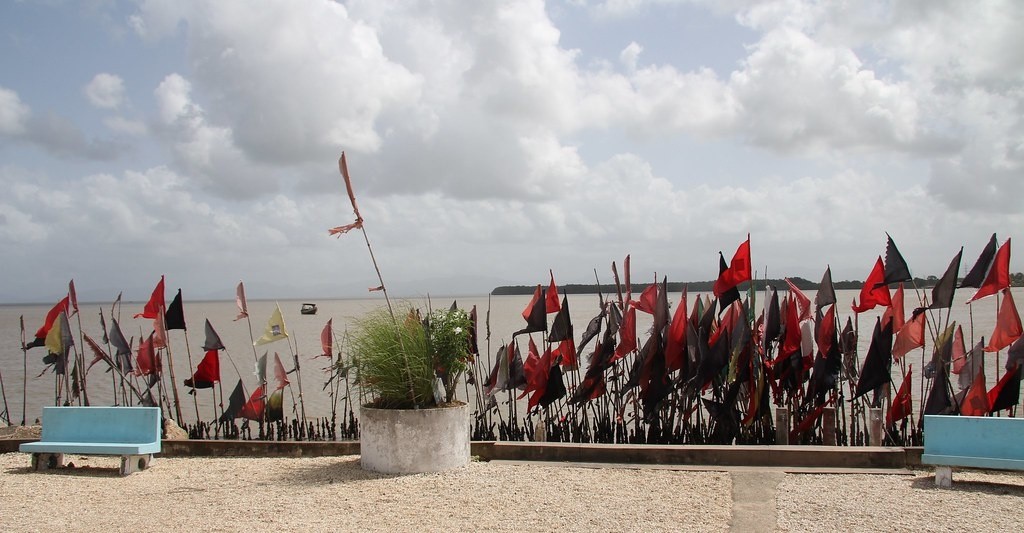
[301,303,318,315]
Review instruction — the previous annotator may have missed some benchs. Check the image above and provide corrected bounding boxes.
[921,415,1024,486]
[19,407,161,474]
